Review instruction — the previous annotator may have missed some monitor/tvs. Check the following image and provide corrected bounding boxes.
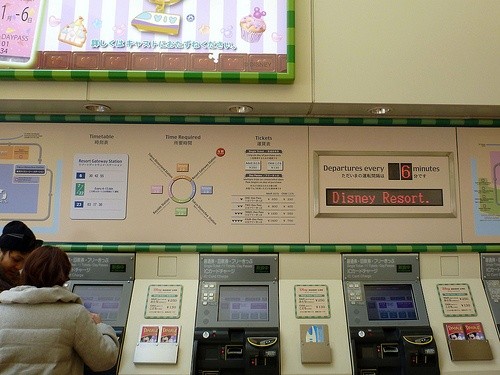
[71,283,124,324]
[217,284,270,322]
[363,283,419,321]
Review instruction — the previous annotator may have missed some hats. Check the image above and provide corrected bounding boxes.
[0,221,44,251]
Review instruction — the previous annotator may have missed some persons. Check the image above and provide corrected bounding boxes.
[0,245,119,375]
[1,219,44,292]
